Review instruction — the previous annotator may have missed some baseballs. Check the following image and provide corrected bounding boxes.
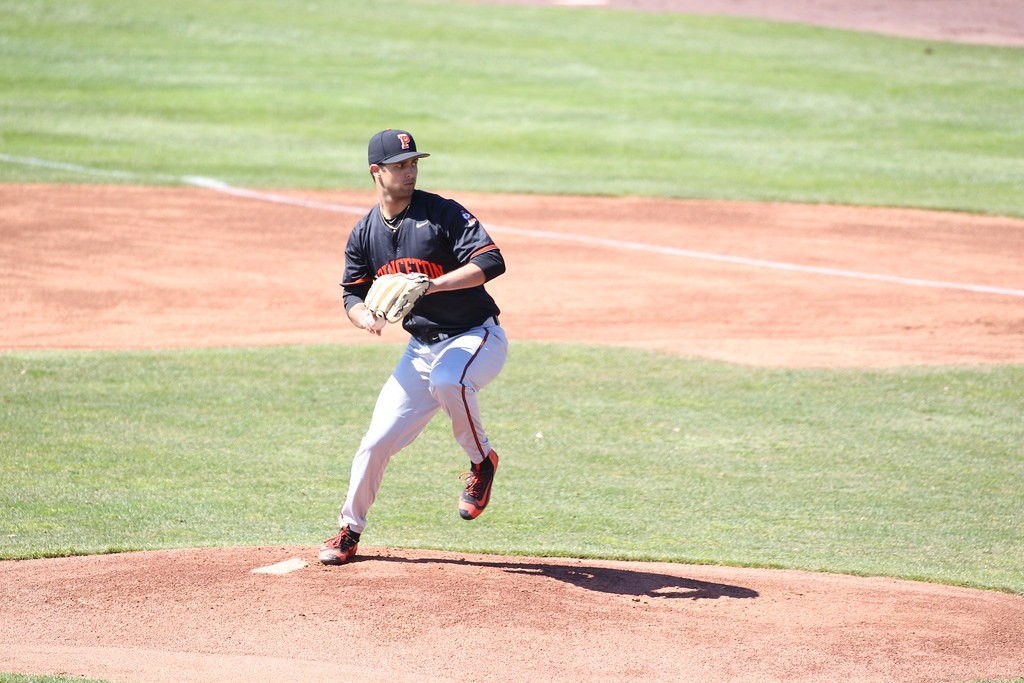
[364,313,385,331]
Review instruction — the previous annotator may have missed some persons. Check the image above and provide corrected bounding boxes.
[317,129,507,561]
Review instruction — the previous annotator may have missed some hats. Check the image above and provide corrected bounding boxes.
[367,128,431,166]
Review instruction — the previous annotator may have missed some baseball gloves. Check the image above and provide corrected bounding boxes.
[363,271,431,324]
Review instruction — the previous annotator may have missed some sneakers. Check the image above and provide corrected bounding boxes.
[318,526,359,566]
[458,449,500,521]
